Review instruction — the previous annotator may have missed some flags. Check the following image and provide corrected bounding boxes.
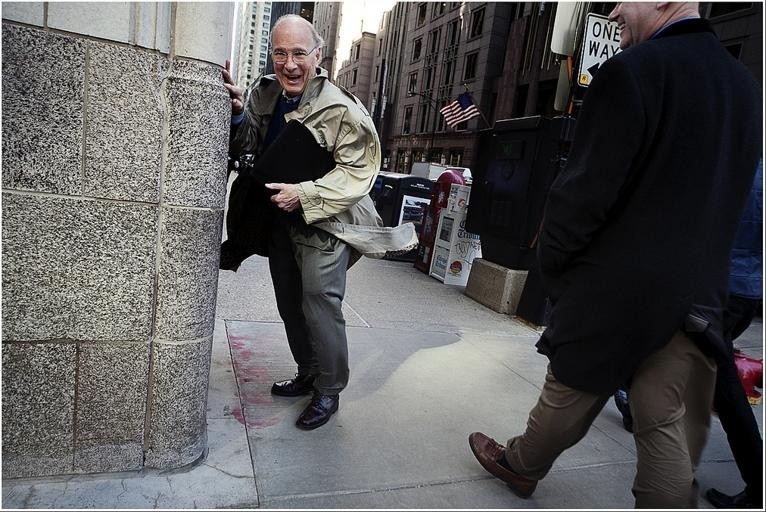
[438,91,480,129]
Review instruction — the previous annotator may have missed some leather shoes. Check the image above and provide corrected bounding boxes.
[705,488,763,509]
[295,393,339,432]
[270,373,315,397]
[468,431,538,499]
[614,389,634,433]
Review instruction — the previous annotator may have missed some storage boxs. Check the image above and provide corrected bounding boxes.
[429,182,482,287]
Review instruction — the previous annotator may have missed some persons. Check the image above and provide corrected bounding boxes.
[465,2,765,510]
[614,159,764,510]
[217,13,383,436]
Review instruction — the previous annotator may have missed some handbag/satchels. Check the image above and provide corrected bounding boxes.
[248,119,337,239]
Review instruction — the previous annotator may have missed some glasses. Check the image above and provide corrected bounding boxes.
[270,47,318,62]
